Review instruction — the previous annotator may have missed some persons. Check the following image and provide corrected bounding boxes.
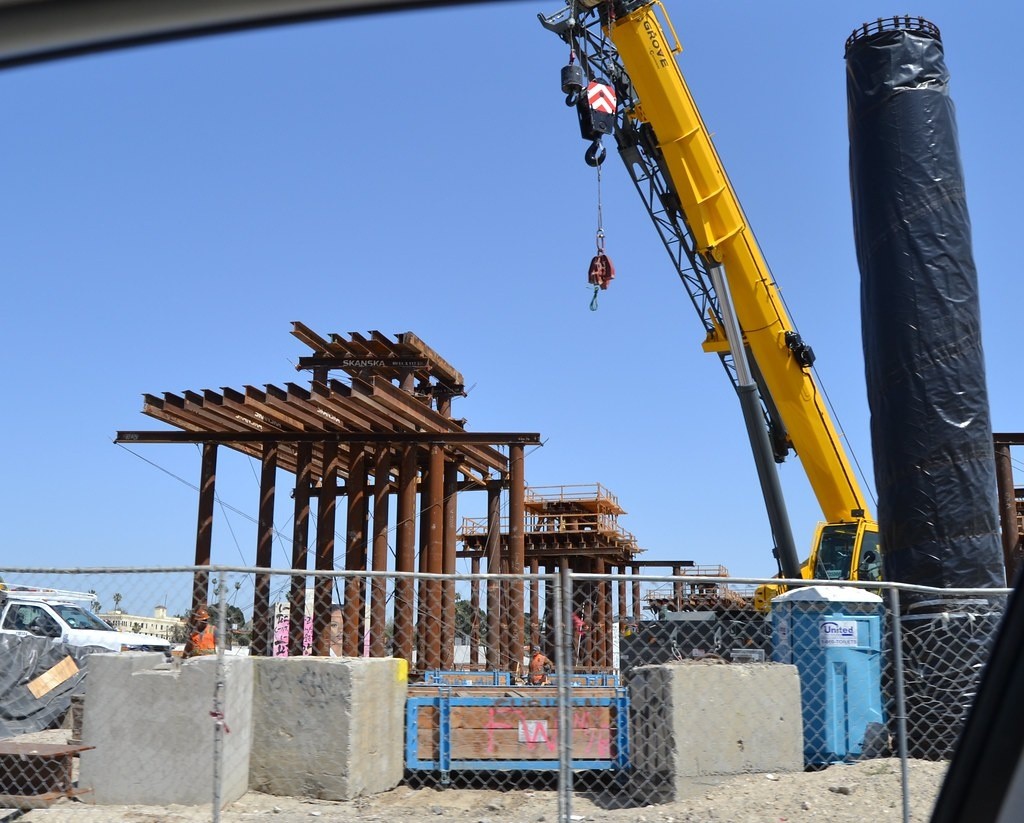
[528,645,554,685]
[180,610,220,659]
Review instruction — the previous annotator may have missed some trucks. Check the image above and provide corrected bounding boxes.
[0,582,172,663]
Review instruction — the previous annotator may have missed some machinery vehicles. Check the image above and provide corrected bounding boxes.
[537,0,896,653]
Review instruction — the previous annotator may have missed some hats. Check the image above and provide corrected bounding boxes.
[533,645,540,651]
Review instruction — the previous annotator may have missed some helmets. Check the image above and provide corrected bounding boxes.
[194,609,210,620]
